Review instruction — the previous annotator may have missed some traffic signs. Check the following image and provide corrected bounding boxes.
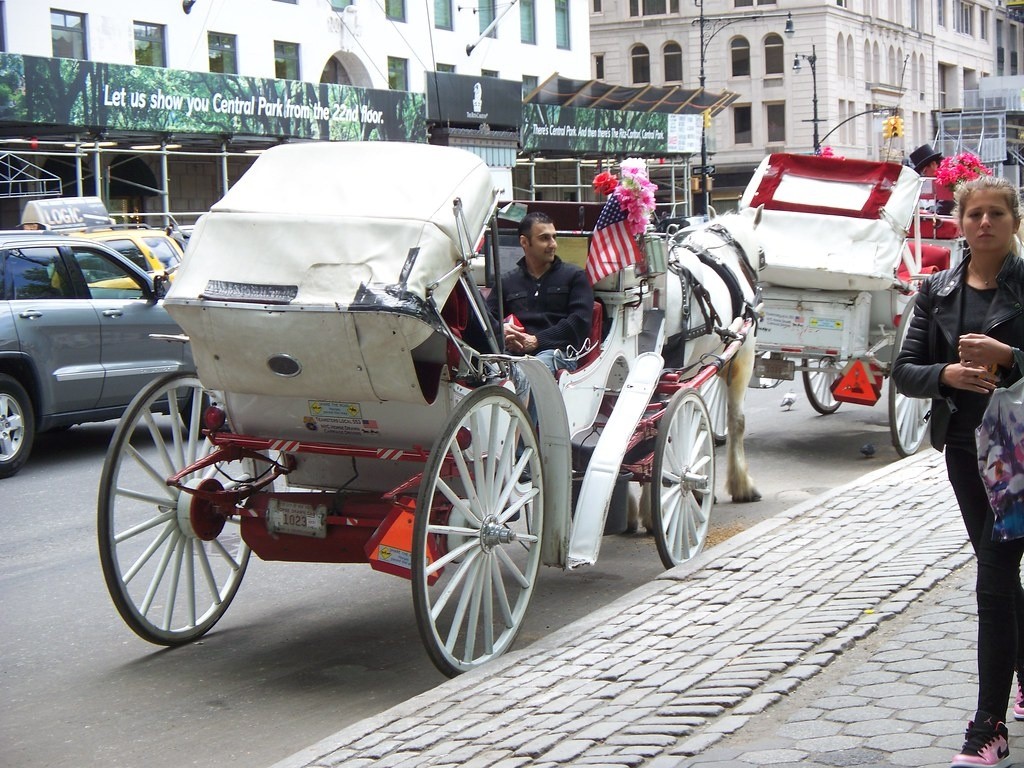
[691,165,716,176]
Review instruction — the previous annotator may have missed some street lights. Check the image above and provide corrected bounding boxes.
[690,1,794,225]
[793,46,821,155]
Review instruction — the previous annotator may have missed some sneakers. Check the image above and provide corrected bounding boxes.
[949,710,1011,768]
[1012,667,1024,718]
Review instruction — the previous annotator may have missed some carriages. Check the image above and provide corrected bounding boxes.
[739,151,969,458]
[97,135,768,674]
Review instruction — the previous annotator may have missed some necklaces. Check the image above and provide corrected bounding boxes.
[970,260,995,285]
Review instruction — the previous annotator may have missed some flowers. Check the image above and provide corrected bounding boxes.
[592,169,657,238]
[937,155,996,191]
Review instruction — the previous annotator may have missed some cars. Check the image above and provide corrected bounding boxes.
[0,198,195,295]
[1,242,210,481]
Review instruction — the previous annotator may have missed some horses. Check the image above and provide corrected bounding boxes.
[616,200,766,535]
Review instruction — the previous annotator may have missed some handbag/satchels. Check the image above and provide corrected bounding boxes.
[974,346,1024,542]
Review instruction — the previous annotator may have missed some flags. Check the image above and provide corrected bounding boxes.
[585,186,641,286]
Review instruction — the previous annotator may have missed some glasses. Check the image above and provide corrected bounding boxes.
[921,167,927,176]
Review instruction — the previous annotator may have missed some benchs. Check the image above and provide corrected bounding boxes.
[478,286,604,380]
[895,220,963,289]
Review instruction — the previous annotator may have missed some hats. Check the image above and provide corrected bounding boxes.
[910,144,942,171]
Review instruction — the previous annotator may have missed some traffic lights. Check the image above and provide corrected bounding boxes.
[882,116,905,139]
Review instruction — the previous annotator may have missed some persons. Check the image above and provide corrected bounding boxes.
[893,176,1024,768]
[485,212,593,483]
[909,143,957,215]
